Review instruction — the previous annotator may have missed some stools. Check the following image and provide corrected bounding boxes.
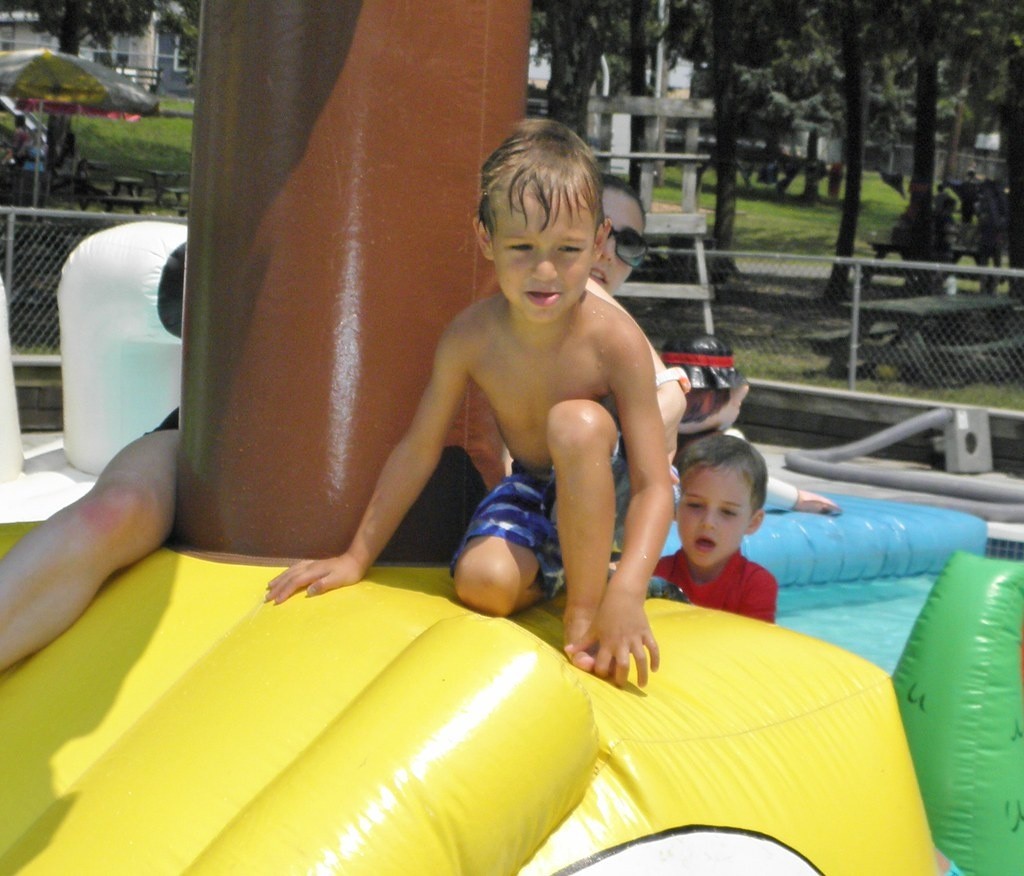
[79,169,189,217]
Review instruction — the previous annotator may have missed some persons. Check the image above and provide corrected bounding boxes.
[265,121,676,686]
[893,169,1014,296]
[2,116,75,207]
[587,172,844,626]
[0,404,179,676]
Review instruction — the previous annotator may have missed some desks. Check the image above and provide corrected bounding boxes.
[825,293,1024,391]
[859,240,989,292]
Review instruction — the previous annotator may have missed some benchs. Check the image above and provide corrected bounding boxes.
[802,325,898,380]
[933,335,1024,390]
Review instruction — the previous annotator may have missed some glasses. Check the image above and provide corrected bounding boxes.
[607,225,650,268]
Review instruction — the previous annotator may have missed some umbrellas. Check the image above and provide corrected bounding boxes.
[1,47,159,215]
